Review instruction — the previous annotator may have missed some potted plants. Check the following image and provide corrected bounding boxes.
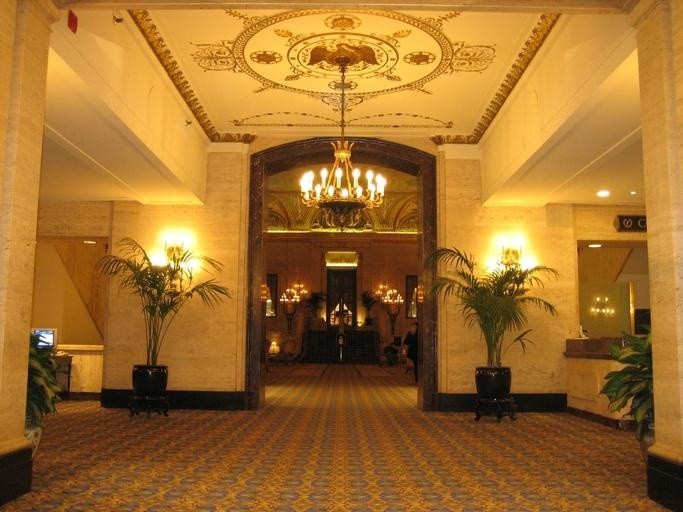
[598,318,653,461]
[414,239,565,397]
[85,234,232,397]
[21,329,76,461]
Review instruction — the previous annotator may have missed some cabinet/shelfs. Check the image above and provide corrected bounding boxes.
[55,342,103,400]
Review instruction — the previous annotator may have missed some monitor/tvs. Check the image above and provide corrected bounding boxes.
[634,308,650,335]
[31,327,57,350]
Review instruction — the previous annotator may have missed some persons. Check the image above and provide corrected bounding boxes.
[402,322,418,384]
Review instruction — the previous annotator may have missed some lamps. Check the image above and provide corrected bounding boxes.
[260,276,423,365]
[287,55,387,232]
